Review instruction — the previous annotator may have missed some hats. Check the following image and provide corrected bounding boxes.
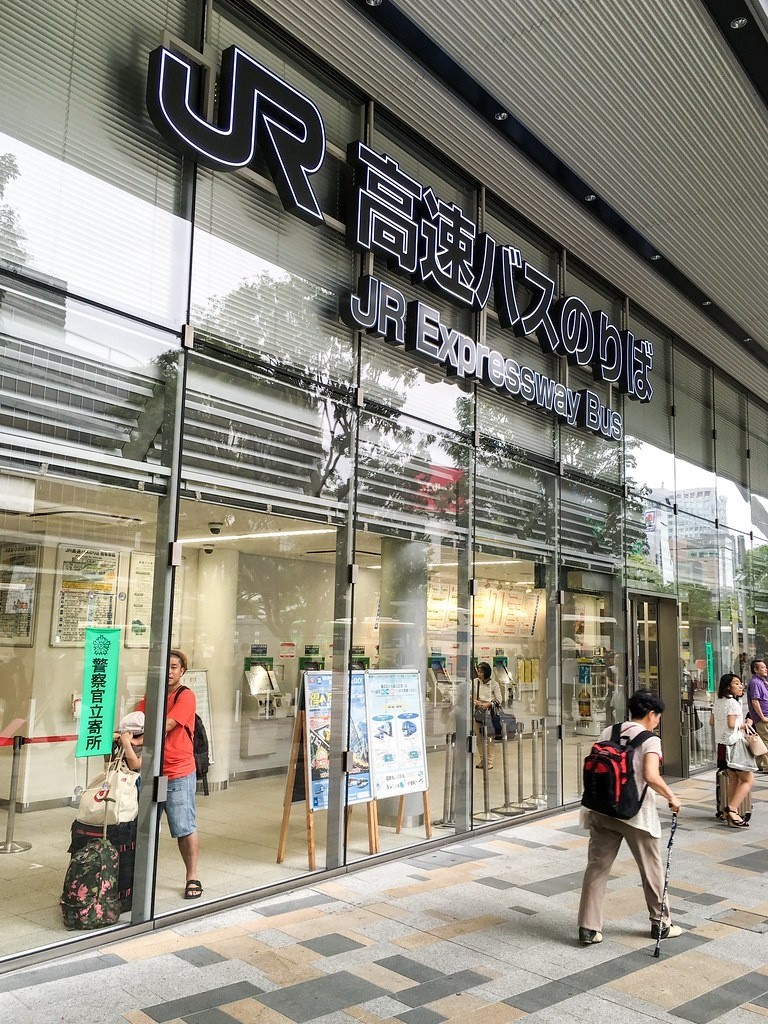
[119,711,145,736]
[171,648,188,677]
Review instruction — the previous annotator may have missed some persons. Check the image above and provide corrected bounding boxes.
[113,711,145,769]
[710,673,755,828]
[605,651,622,726]
[134,649,203,900]
[563,651,577,720]
[470,662,503,770]
[710,651,747,693]
[454,682,467,786]
[577,688,682,946]
[679,656,704,752]
[747,659,768,771]
[508,690,513,708]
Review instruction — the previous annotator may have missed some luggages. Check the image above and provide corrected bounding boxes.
[716,767,752,821]
[69,818,137,914]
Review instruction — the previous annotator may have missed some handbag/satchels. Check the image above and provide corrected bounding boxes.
[727,724,759,773]
[475,698,516,742]
[745,723,768,757]
[79,758,138,826]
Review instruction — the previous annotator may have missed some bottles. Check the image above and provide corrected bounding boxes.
[579,686,590,727]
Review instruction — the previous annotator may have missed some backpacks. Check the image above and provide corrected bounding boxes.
[582,723,661,820]
[174,685,211,780]
[59,839,121,931]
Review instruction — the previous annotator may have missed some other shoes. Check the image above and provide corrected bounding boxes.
[652,924,682,939]
[476,761,483,768]
[487,762,493,770]
[579,927,602,943]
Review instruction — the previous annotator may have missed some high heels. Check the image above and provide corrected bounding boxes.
[719,807,750,828]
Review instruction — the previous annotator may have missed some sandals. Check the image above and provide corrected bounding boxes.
[185,879,203,899]
